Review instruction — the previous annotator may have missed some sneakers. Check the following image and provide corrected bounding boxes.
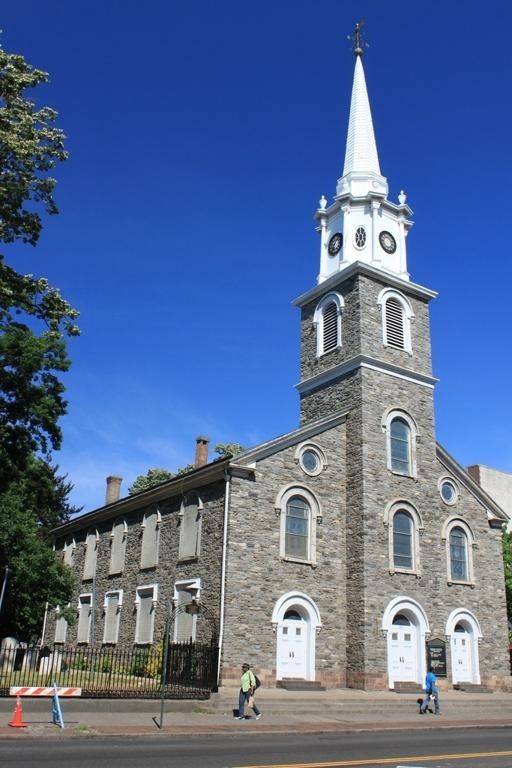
[256,714,262,720]
[234,717,246,720]
[435,712,442,715]
[420,709,426,714]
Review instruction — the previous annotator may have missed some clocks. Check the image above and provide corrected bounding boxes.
[328,232,343,256]
[379,230,397,255]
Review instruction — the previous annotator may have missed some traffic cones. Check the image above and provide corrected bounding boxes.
[8,694,31,729]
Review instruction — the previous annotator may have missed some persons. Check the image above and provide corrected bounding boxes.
[418,665,443,715]
[235,662,261,720]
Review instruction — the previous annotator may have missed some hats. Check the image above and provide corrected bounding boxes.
[241,663,250,668]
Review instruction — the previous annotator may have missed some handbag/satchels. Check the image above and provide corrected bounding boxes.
[249,672,260,691]
